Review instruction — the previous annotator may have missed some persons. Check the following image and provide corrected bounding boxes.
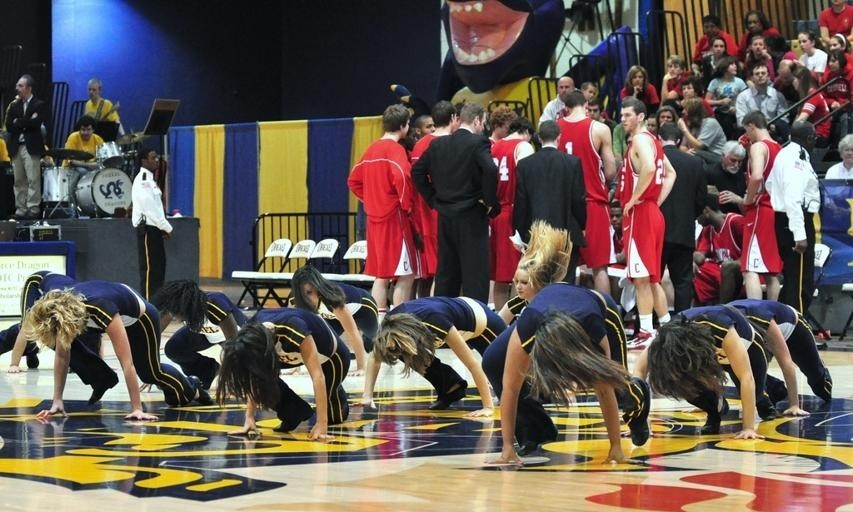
[481,283,651,464]
[216,308,350,442]
[349,296,507,417]
[131,147,173,302]
[346,0,853,350]
[24,279,213,420]
[140,280,247,408]
[4,73,126,221]
[631,298,833,439]
[281,266,378,377]
[0,270,103,373]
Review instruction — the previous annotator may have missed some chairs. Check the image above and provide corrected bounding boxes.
[813,243,832,339]
[840,283,852,339]
[305,238,336,279]
[258,240,314,308]
[231,238,290,308]
[337,241,376,284]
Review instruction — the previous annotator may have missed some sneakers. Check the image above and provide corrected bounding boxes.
[273,403,314,432]
[701,400,729,433]
[190,376,213,403]
[758,407,777,421]
[768,386,787,407]
[202,358,221,390]
[517,427,558,456]
[25,212,39,218]
[26,346,40,368]
[88,373,118,406]
[429,387,466,409]
[338,384,349,421]
[9,214,22,220]
[626,327,652,349]
[630,383,650,445]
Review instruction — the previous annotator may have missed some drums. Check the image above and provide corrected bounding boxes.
[95,141,120,167]
[74,168,132,216]
[42,167,80,203]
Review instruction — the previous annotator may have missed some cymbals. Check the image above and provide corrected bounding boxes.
[45,149,94,160]
[115,132,150,144]
[103,156,120,167]
